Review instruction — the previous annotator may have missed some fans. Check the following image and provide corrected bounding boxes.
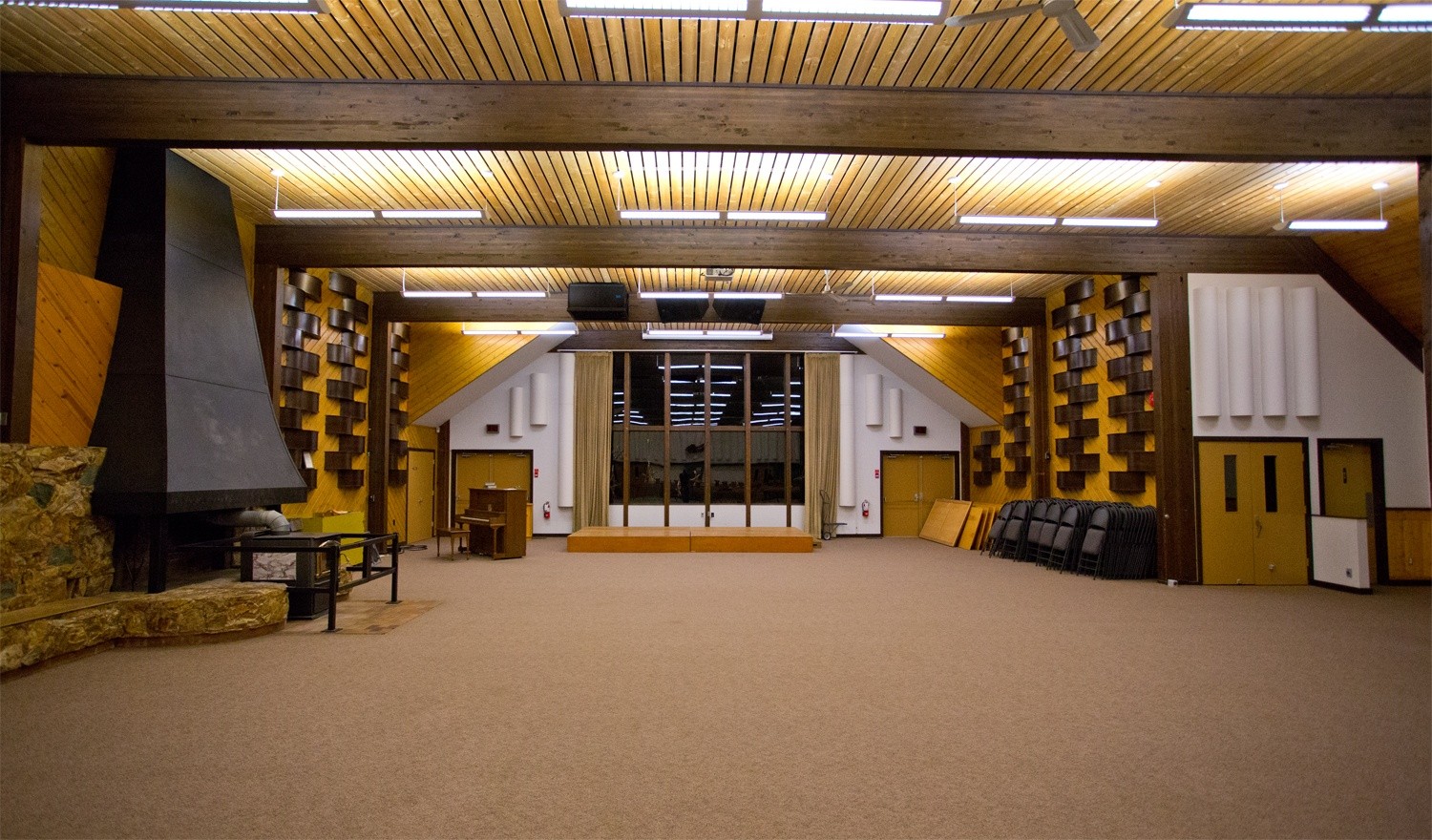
[945,1,1100,53]
[799,270,854,304]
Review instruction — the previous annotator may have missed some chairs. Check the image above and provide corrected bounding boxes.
[979,498,1160,581]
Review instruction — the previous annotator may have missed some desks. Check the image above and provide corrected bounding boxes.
[241,533,398,634]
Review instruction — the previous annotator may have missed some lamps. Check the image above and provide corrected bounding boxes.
[556,0,949,25]
[613,168,834,222]
[642,323,763,338]
[1161,0,1432,34]
[462,321,579,336]
[635,268,786,303]
[948,177,1162,227]
[0,0,331,15]
[270,168,493,222]
[1272,182,1388,230]
[871,269,1016,303]
[830,324,946,340]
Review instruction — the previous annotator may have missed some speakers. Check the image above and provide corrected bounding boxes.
[566,283,627,313]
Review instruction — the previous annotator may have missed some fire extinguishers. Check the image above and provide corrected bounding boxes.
[862,500,869,516]
[543,501,550,518]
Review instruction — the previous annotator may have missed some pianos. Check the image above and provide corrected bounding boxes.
[454,487,527,560]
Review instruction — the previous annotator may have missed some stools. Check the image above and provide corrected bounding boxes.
[435,527,472,560]
